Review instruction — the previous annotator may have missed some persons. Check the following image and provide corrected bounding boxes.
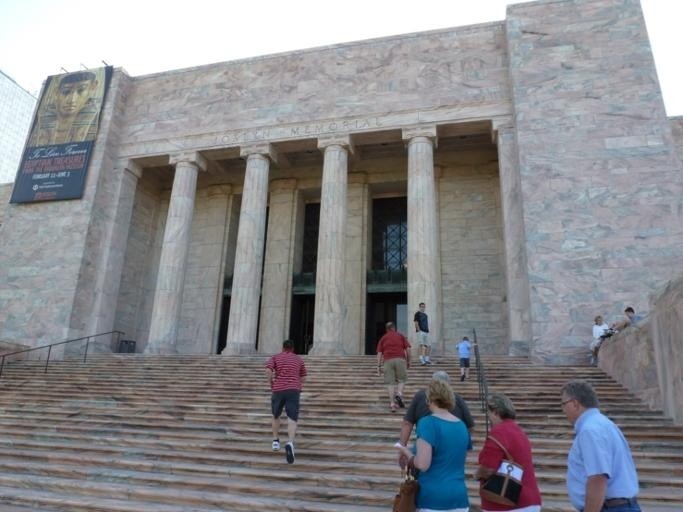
[266,341,307,463]
[28,73,102,145]
[589,315,609,365]
[605,307,643,333]
[473,393,542,512]
[456,337,478,382]
[397,371,475,469]
[376,322,411,412]
[395,379,472,512]
[414,303,433,365]
[561,379,641,512]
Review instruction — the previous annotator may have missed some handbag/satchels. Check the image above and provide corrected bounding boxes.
[480,436,524,506]
[393,455,419,512]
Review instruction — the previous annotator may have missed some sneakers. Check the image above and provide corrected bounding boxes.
[391,394,405,411]
[420,361,430,365]
[461,374,469,380]
[285,442,294,463]
[272,442,280,450]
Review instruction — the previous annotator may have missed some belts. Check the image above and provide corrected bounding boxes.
[606,500,629,507]
